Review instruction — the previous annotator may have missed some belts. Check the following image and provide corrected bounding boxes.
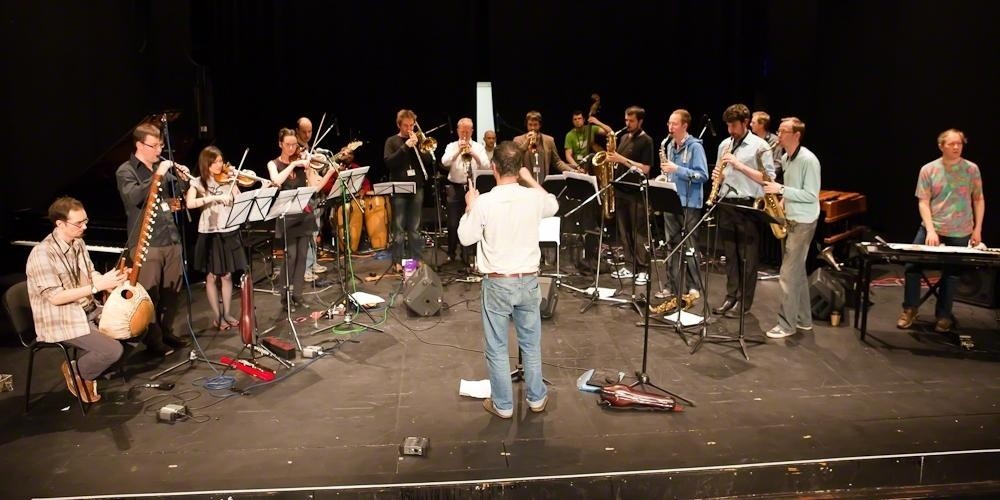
[483,272,537,279]
[716,196,755,202]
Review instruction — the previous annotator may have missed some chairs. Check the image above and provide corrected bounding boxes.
[1,278,97,418]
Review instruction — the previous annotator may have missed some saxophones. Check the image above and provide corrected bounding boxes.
[592,126,630,219]
[655,132,673,182]
[640,293,696,314]
[753,140,788,239]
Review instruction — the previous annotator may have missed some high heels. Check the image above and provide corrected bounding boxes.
[281,295,313,313]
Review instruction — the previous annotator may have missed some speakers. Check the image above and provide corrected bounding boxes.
[808,268,846,320]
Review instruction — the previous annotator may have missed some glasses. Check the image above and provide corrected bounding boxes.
[775,129,794,136]
[57,218,89,229]
[282,142,299,148]
[666,122,678,126]
[141,140,166,151]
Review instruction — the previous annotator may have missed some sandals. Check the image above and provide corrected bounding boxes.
[212,320,241,331]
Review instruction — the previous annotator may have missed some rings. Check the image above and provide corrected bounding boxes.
[935,243,940,246]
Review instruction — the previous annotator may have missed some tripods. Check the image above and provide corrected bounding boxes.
[373,190,410,285]
[583,245,696,408]
[254,214,319,354]
[546,174,737,353]
[219,222,291,370]
[308,189,385,333]
[708,227,767,362]
[442,189,484,287]
[509,346,553,386]
[422,161,455,273]
[149,189,240,381]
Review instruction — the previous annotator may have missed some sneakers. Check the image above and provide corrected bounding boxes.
[765,323,796,339]
[304,271,319,283]
[654,288,675,299]
[796,321,814,330]
[610,267,634,279]
[934,318,955,333]
[634,271,650,286]
[165,331,188,348]
[896,305,920,330]
[311,263,328,274]
[688,287,701,299]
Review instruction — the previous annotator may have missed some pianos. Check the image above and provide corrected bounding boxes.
[855,241,1000,265]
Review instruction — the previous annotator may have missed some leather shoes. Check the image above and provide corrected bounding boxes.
[482,399,513,421]
[712,298,734,316]
[62,359,102,403]
[724,300,750,319]
[531,395,549,412]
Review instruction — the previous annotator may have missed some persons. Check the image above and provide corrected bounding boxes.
[458,141,559,418]
[26,197,132,403]
[897,128,986,331]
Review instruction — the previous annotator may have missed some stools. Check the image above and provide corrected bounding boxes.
[908,266,948,321]
[235,229,275,290]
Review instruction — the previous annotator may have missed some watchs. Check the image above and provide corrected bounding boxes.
[90,282,98,294]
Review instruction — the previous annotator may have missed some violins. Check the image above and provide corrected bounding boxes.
[296,152,340,169]
[214,161,282,190]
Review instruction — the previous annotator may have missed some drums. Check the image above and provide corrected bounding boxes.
[337,192,364,255]
[365,190,392,249]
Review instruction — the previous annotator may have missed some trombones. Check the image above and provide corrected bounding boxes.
[408,121,437,181]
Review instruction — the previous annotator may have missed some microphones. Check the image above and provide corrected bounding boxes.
[316,147,330,154]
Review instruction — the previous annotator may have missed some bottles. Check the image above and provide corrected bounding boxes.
[831,311,841,328]
